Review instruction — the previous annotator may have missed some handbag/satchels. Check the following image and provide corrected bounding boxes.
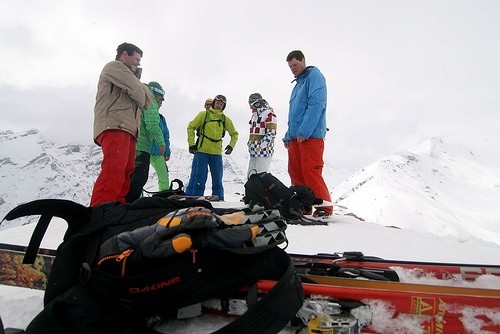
[240,168,304,220]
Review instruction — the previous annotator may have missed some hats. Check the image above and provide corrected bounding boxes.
[147,81,164,97]
[204,98,213,108]
[248,93,263,109]
[215,95,227,103]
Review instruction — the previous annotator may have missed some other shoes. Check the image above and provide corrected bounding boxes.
[313,209,330,219]
[301,206,312,215]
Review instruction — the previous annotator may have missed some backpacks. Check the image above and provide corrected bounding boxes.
[1,178,306,333]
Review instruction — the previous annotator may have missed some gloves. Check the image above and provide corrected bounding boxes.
[224,145,232,155]
[188,145,198,154]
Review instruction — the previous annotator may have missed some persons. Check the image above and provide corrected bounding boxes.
[184,95,238,201]
[247,93,276,177]
[88,43,152,208]
[282,50,334,218]
[124,81,171,203]
